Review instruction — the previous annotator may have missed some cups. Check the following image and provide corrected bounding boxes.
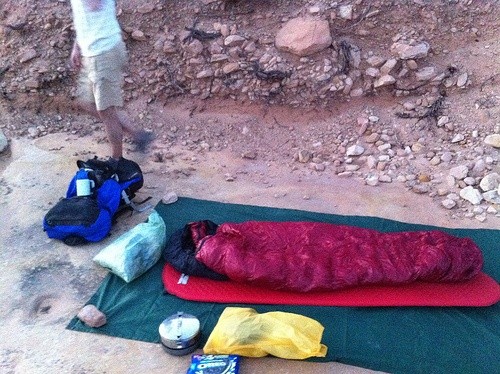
[76,179,95,196]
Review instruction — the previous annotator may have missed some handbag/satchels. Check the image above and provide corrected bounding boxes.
[92,209,167,283]
[202,306,328,359]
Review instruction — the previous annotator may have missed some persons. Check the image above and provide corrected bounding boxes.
[68,0,156,161]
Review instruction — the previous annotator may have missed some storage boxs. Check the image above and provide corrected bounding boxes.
[159,311,201,356]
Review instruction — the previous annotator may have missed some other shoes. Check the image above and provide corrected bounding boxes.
[135,130,150,152]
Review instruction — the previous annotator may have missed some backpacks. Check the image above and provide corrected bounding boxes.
[43,157,153,246]
